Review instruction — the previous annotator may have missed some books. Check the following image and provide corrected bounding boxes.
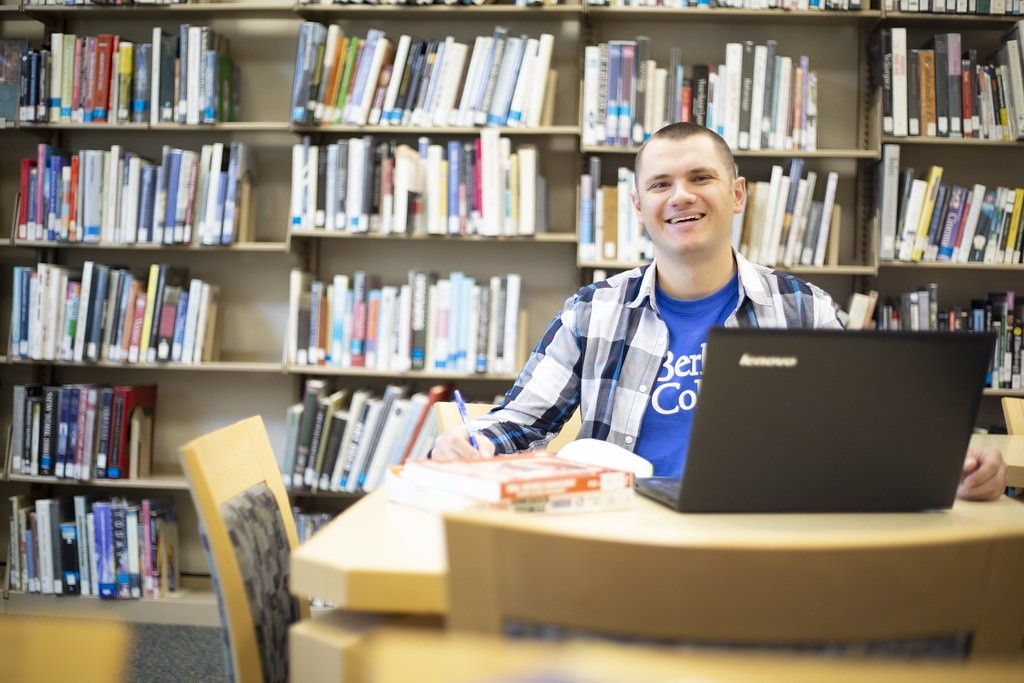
[384,464,635,519]
[402,450,632,504]
[288,0,1024,544]
[9,0,256,598]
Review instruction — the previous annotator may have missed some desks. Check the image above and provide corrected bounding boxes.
[289,455,1024,683]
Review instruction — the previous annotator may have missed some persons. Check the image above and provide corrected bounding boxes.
[433,122,1006,501]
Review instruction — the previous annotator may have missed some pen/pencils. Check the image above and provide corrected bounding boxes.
[454,389,477,449]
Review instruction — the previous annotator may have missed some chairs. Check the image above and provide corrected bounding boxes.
[184,419,309,683]
[439,510,1021,683]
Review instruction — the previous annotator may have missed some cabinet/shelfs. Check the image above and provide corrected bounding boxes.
[0,0,1024,607]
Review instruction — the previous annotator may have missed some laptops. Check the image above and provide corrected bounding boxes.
[633,327,998,513]
